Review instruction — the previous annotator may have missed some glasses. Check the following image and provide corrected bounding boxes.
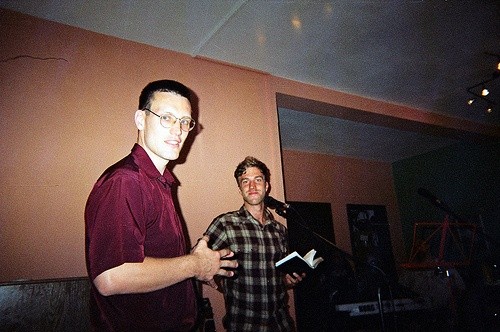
[142,108,196,132]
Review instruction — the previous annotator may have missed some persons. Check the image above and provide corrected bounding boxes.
[84,80,239,332]
[191,156,306,332]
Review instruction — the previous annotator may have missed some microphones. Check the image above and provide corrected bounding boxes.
[264,196,292,209]
[417,188,447,210]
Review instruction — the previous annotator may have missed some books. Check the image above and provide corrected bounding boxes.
[275,249,324,279]
